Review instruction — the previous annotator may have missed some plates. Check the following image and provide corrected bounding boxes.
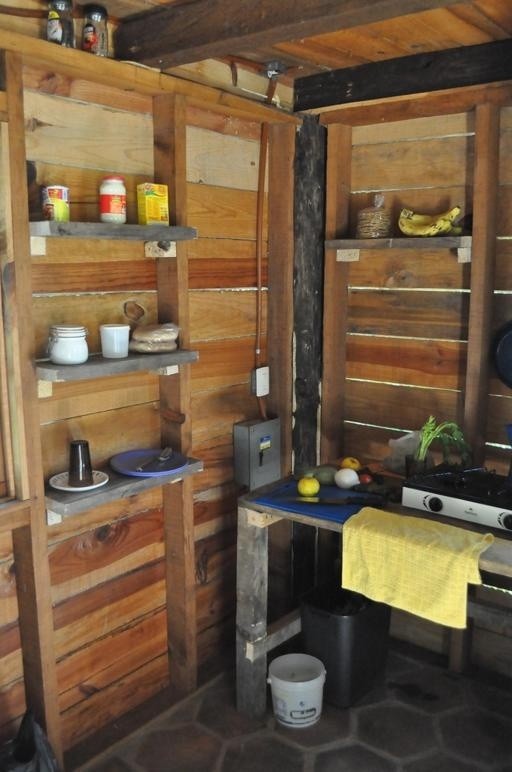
[48,470,110,492]
[110,448,189,477]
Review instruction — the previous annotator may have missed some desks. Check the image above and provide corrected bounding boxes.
[235,464,511,717]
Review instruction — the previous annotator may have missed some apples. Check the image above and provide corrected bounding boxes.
[298,476,321,496]
[341,457,360,472]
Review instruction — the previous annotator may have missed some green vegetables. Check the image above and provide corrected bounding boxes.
[413,415,473,466]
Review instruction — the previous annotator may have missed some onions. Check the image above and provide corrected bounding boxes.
[335,467,360,490]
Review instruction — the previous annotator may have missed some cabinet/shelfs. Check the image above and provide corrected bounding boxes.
[30,220,203,524]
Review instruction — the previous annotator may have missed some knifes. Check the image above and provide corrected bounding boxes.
[288,496,387,509]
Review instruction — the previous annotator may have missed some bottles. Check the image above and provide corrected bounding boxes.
[81,3,110,59]
[45,323,90,366]
[26,160,45,222]
[46,0,76,50]
[98,175,128,224]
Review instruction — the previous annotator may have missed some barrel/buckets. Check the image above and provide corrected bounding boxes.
[267,653,327,731]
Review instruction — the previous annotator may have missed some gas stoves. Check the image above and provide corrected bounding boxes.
[400,461,512,531]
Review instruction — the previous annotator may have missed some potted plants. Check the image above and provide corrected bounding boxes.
[406,416,471,480]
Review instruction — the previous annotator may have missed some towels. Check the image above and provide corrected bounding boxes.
[341,506,494,630]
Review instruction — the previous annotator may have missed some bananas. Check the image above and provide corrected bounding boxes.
[399,205,466,236]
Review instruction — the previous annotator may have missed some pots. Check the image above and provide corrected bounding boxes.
[490,321,512,389]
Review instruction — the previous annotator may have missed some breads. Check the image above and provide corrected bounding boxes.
[129,323,179,353]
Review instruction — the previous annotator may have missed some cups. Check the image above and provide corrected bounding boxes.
[99,323,131,359]
[67,439,94,487]
